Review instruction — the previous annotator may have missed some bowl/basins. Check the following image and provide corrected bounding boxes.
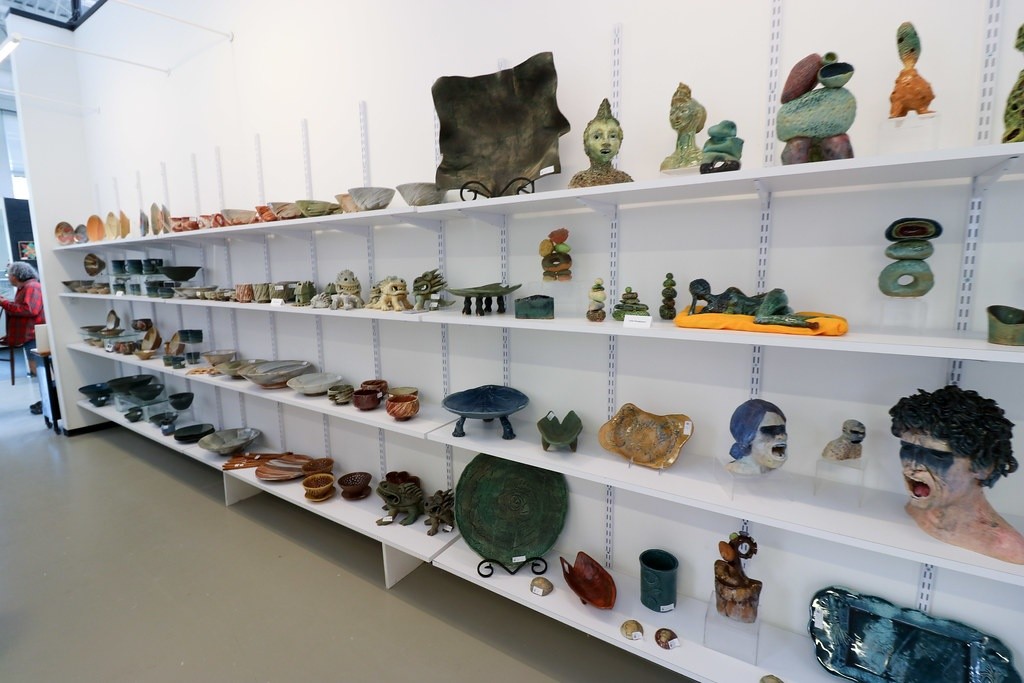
[168,392,194,410]
[267,201,302,220]
[149,412,178,427]
[386,386,418,396]
[157,265,201,281]
[89,394,110,407]
[396,182,447,206]
[107,374,153,392]
[215,358,267,379]
[131,383,165,401]
[348,187,396,211]
[198,427,261,456]
[386,394,420,421]
[352,389,382,411]
[221,208,257,224]
[78,382,112,400]
[327,384,354,405]
[125,410,142,422]
[200,350,236,367]
[61,280,95,293]
[360,379,388,396]
[286,372,342,397]
[334,194,361,213]
[171,286,217,298]
[255,205,277,222]
[296,200,340,218]
[237,360,312,389]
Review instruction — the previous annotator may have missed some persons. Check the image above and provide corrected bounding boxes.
[887,383,1024,564]
[661,83,706,170]
[726,398,788,476]
[0,261,46,414]
[567,99,632,188]
[822,420,867,460]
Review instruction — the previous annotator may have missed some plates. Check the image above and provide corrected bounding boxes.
[55,203,171,246]
[174,423,215,443]
[455,453,569,567]
[444,282,522,297]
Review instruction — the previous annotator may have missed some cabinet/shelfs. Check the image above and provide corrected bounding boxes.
[48,143,1024,683]
[30,349,61,436]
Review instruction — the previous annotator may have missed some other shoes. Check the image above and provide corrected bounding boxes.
[30,401,41,409]
[32,406,42,414]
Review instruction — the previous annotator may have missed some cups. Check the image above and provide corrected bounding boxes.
[111,259,163,298]
[639,549,679,613]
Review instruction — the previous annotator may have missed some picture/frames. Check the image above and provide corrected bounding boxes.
[18,240,36,261]
